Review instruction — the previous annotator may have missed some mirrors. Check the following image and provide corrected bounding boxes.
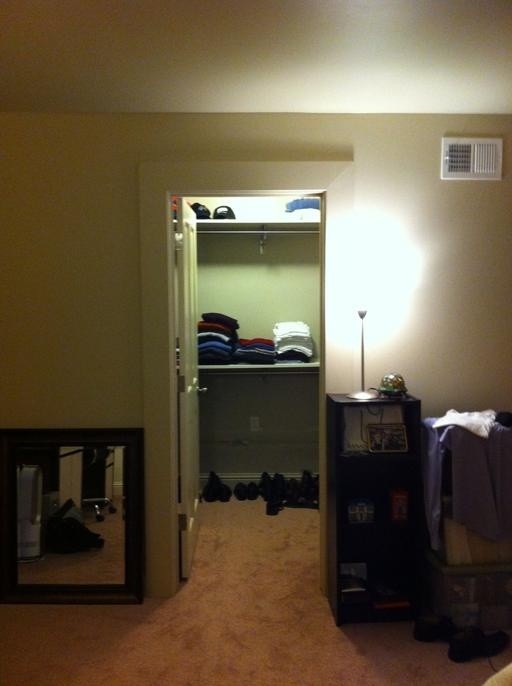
[0,428,145,607]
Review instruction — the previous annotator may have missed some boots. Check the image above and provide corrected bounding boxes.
[203,471,231,502]
[235,471,319,516]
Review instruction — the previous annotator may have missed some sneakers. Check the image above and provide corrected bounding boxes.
[414,616,506,662]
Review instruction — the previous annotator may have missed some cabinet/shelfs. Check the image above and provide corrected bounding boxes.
[325,390,512,652]
[172,216,322,495]
